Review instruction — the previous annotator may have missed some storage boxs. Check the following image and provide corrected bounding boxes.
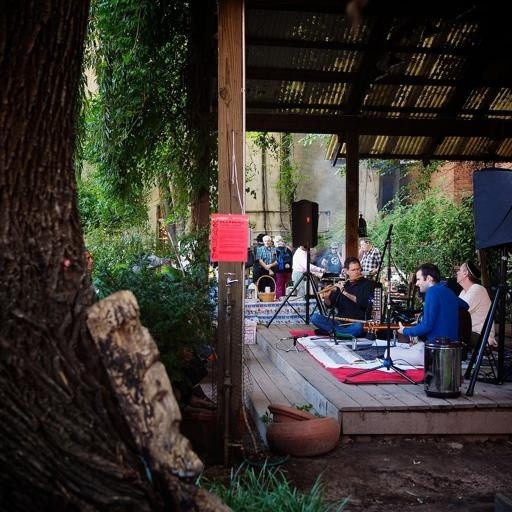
[244,320,258,344]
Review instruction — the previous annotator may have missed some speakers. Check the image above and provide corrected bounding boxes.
[474,167,512,249]
[292,199,318,248]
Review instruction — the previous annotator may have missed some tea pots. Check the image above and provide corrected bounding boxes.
[284,281,297,296]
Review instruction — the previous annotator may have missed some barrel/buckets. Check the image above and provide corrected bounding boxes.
[424,342,463,396]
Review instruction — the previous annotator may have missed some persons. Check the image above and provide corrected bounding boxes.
[253,234,278,292]
[254,233,265,255]
[357,237,386,280]
[291,245,327,296]
[316,240,342,274]
[456,261,496,348]
[311,256,383,339]
[274,235,292,301]
[383,263,460,369]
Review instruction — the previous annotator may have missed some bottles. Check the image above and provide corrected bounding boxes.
[358,213,367,238]
[423,335,462,399]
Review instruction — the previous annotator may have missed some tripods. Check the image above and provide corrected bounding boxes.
[265,248,327,329]
[347,224,418,385]
[310,288,352,345]
[286,338,299,354]
[464,249,512,396]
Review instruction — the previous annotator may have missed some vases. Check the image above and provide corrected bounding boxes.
[266,415,341,457]
[267,402,316,423]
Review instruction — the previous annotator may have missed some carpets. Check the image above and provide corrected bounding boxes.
[288,329,427,384]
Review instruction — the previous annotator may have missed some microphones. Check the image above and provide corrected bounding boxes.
[367,296,373,312]
[281,333,308,340]
[343,277,350,288]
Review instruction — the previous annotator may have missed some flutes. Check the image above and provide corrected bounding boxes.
[318,274,365,295]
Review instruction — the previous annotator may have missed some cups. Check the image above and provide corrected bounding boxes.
[245,289,255,299]
[265,287,270,293]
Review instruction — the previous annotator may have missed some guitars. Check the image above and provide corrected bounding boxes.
[364,311,472,343]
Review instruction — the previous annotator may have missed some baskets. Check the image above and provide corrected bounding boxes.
[256,274,277,303]
